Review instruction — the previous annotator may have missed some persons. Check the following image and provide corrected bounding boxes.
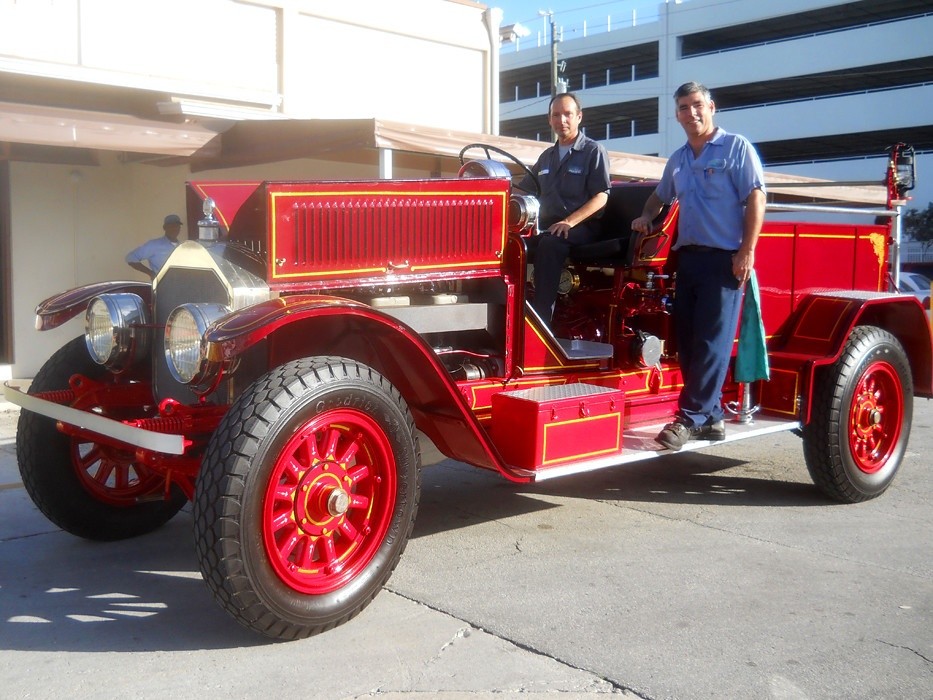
[631,82,767,451]
[126,214,184,283]
[512,91,614,326]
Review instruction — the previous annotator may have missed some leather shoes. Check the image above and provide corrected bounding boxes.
[655,422,691,450]
[689,420,725,440]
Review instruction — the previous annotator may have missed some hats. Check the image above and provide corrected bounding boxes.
[164,215,183,226]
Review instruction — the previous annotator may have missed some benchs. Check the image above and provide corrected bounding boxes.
[567,184,679,307]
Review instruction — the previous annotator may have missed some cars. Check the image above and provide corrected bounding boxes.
[0,142,933,643]
[887,271,933,325]
